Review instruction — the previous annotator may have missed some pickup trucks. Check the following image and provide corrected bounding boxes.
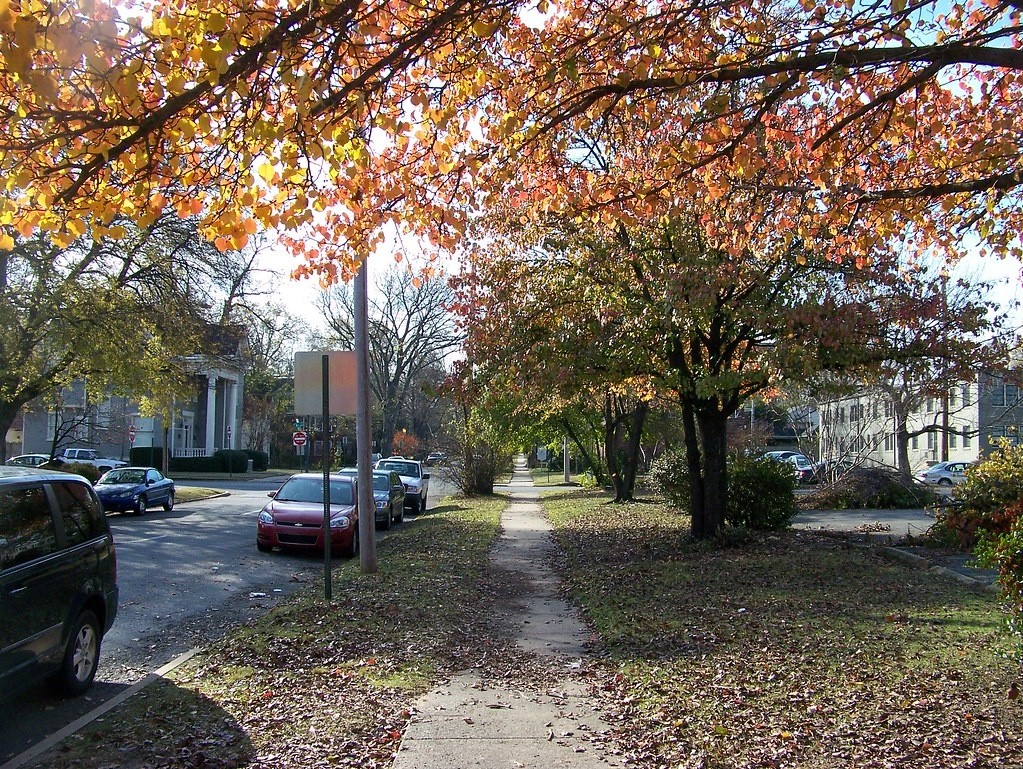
[57,448,128,474]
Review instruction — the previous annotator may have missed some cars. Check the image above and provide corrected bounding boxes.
[355,453,383,468]
[473,455,482,461]
[375,459,430,513]
[5,452,57,468]
[755,451,801,463]
[796,460,878,483]
[915,461,977,485]
[426,453,443,466]
[93,467,175,515]
[385,456,408,472]
[442,453,448,465]
[788,455,814,479]
[0,466,118,698]
[337,467,405,529]
[257,473,377,555]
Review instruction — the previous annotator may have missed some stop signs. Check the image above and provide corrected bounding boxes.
[227,425,232,439]
[293,432,307,445]
[129,426,136,442]
